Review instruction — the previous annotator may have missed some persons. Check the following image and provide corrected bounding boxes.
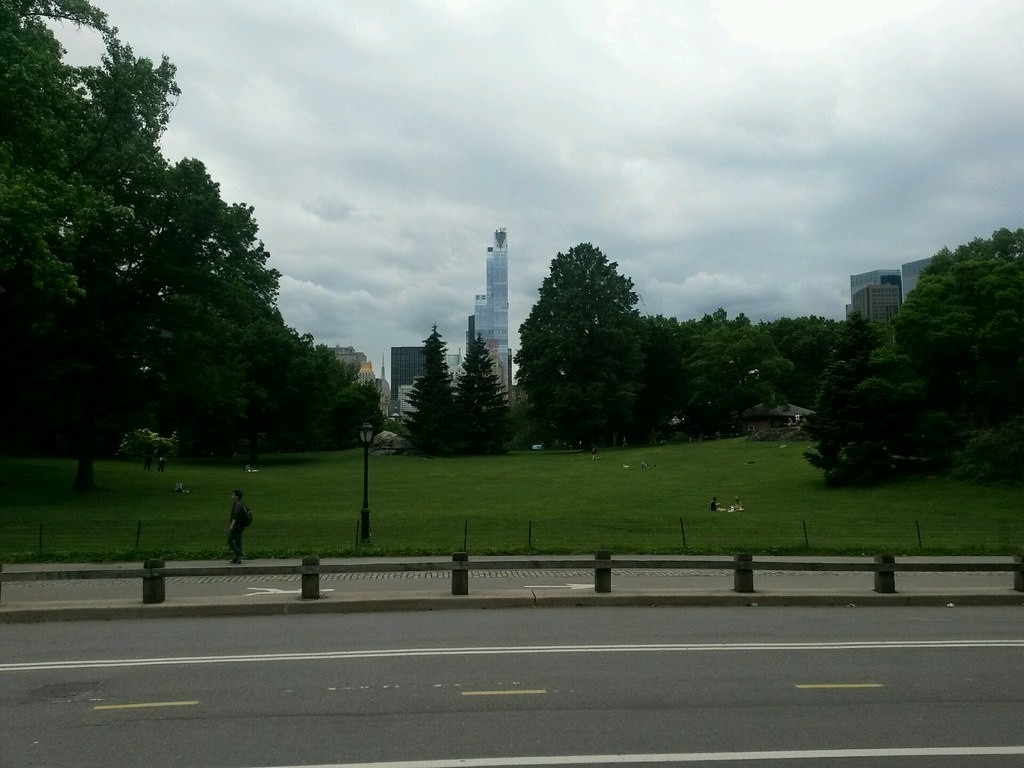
[710,496,720,510]
[734,495,742,509]
[788,417,792,426]
[699,424,756,441]
[623,436,627,447]
[796,412,801,421]
[592,447,599,460]
[227,489,247,565]
[661,439,665,444]
[143,451,167,472]
[689,433,692,442]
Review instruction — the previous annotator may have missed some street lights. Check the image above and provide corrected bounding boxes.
[357,420,376,543]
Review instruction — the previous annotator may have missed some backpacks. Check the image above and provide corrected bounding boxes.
[240,506,253,526]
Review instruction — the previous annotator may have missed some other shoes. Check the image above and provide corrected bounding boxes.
[229,560,244,564]
[236,553,246,563]
